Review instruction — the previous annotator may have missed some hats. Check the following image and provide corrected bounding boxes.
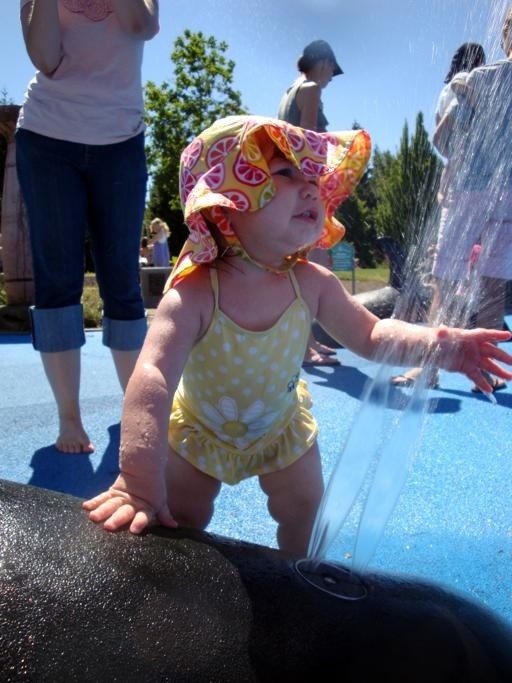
[298,39,344,75]
[162,115,371,294]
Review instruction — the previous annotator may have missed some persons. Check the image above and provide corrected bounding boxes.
[277,36,341,368]
[12,0,160,455]
[81,110,511,564]
[147,215,173,267]
[138,233,153,265]
